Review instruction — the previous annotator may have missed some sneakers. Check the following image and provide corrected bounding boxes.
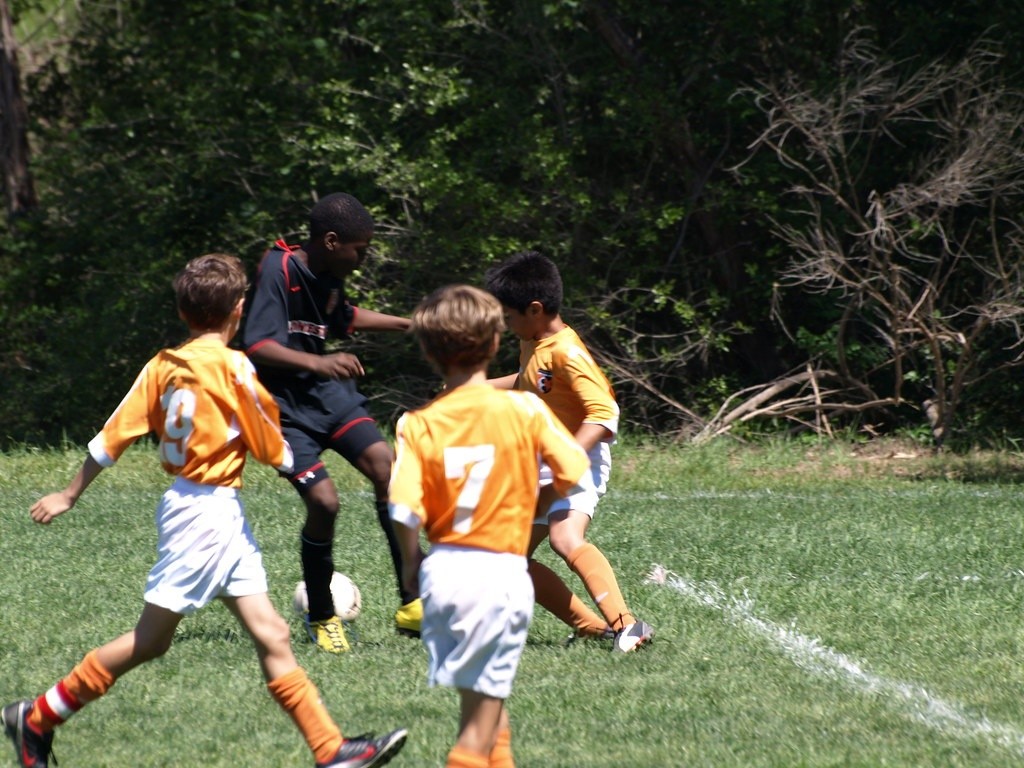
[567,625,614,642]
[0,698,58,768]
[395,598,424,639]
[613,613,656,653]
[315,727,408,768]
[304,609,350,653]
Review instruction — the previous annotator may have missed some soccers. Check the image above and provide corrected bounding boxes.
[294,571,362,622]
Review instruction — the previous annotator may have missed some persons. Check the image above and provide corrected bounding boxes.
[0,253,407,768]
[245,192,424,652]
[388,285,599,768]
[482,246,654,660]
